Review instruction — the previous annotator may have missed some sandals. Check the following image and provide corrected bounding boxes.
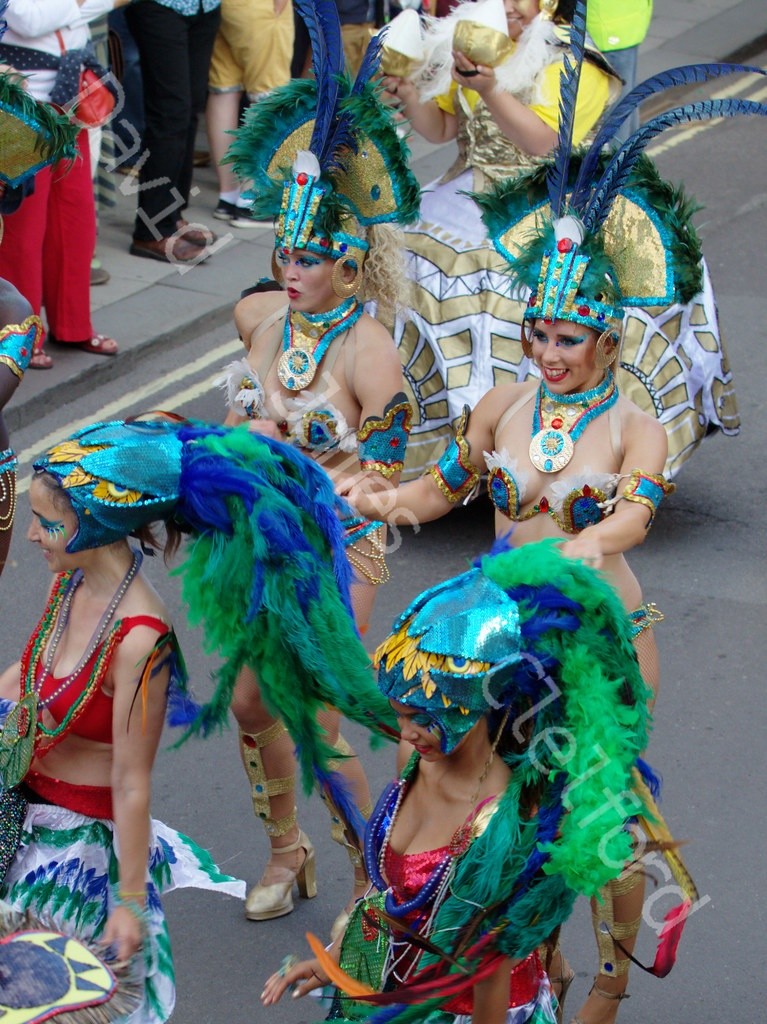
[81,333,119,355]
[27,348,52,369]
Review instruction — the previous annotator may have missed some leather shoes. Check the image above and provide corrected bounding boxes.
[129,218,219,263]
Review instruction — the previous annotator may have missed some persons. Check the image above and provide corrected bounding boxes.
[0,409,248,1023]
[231,161,419,921]
[0,0,421,572]
[348,246,676,1024]
[258,537,653,1024]
[367,0,732,503]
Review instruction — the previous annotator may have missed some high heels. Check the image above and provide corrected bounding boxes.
[245,828,315,920]
[548,969,575,1024]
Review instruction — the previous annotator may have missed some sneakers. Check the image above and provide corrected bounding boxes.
[212,195,281,230]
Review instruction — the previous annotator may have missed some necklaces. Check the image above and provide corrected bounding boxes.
[364,764,507,920]
[19,547,144,762]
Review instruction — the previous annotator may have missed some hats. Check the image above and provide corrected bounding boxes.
[455,0,766,342]
[376,537,646,1007]
[34,412,407,831]
[221,0,421,269]
